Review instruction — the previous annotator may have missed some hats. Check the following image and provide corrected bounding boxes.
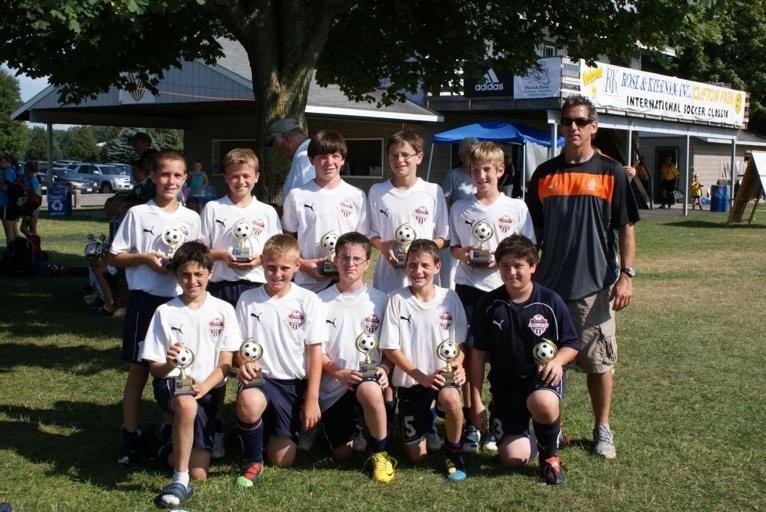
[260,117,301,147]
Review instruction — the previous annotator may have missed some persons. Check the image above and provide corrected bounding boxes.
[86,197,114,308]
[140,240,234,508]
[131,160,143,190]
[96,202,137,313]
[138,149,160,199]
[450,142,535,314]
[133,132,151,160]
[317,232,396,483]
[283,131,370,291]
[690,174,704,210]
[368,131,449,292]
[116,152,203,465]
[273,119,316,213]
[91,189,134,313]
[441,138,481,200]
[658,155,680,209]
[232,234,324,488]
[524,97,641,460]
[202,148,283,302]
[19,161,42,247]
[623,165,637,181]
[379,240,468,481]
[0,154,18,254]
[189,162,209,209]
[468,234,580,485]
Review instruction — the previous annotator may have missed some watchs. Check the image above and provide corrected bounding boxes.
[620,267,636,278]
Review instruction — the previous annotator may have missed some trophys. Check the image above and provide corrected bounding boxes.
[231,222,252,261]
[318,232,341,275]
[161,229,182,267]
[470,220,493,267]
[170,347,200,395]
[394,224,415,266]
[436,340,460,386]
[354,333,380,381]
[239,339,266,388]
[533,339,560,391]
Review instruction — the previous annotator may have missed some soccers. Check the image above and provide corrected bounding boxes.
[164,228,181,244]
[536,342,554,360]
[323,235,338,250]
[234,222,251,240]
[440,342,456,359]
[359,333,375,351]
[173,349,191,366]
[397,226,413,242]
[241,342,259,361]
[475,223,490,239]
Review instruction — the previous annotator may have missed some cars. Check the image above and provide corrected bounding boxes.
[53,168,98,193]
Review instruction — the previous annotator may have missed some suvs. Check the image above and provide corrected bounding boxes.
[69,163,133,193]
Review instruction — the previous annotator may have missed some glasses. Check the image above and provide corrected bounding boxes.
[390,153,417,160]
[337,255,367,265]
[560,116,593,126]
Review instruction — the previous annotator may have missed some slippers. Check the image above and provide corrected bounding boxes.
[156,482,194,509]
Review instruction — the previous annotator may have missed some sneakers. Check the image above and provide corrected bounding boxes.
[116,425,139,464]
[441,440,469,482]
[360,449,398,484]
[461,425,483,454]
[209,426,226,459]
[592,424,617,460]
[537,453,566,484]
[235,459,265,488]
[426,425,445,452]
[482,432,499,458]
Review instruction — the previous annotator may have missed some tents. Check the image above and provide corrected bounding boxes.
[426,119,564,189]
